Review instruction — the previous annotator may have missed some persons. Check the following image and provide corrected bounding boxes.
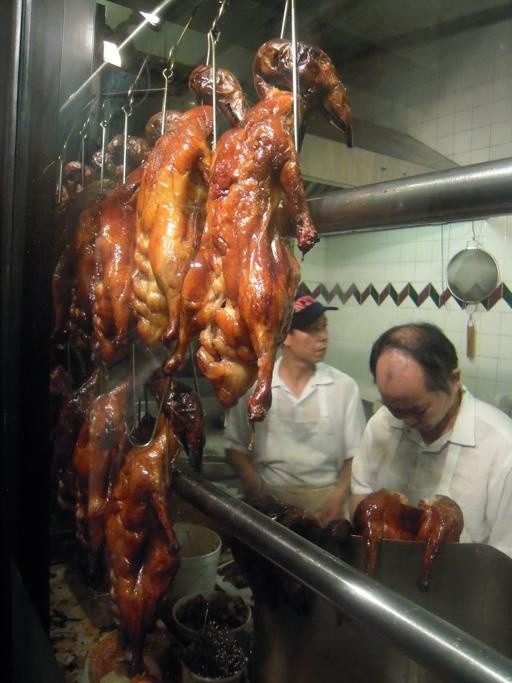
[222,289,365,534]
[348,319,512,563]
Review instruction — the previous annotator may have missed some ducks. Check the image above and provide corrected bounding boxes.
[409,490,465,593]
[354,487,412,578]
[41,31,357,423]
[41,362,209,683]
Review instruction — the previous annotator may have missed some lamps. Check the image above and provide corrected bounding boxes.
[102,31,134,69]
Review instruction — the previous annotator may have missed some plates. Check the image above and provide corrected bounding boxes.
[84,629,178,682]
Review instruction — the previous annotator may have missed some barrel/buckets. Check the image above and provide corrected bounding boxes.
[172,591,252,645]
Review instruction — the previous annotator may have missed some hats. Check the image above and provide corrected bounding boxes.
[289,293,339,331]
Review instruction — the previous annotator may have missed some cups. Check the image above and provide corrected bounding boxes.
[172,590,254,681]
